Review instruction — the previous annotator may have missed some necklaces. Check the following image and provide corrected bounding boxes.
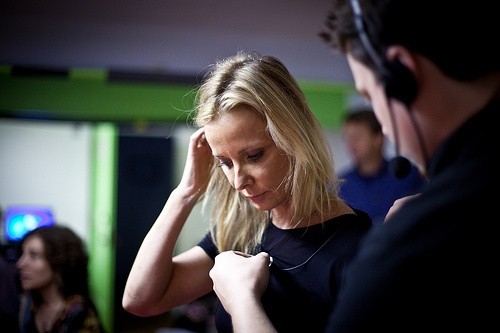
[268,235,336,271]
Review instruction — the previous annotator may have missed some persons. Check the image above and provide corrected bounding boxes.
[16,226,101,333]
[334,110,425,225]
[121,49,373,333]
[208,0,500,333]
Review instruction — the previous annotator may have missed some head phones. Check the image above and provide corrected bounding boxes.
[350,0,418,107]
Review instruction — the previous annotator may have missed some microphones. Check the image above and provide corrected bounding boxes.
[387,95,412,181]
